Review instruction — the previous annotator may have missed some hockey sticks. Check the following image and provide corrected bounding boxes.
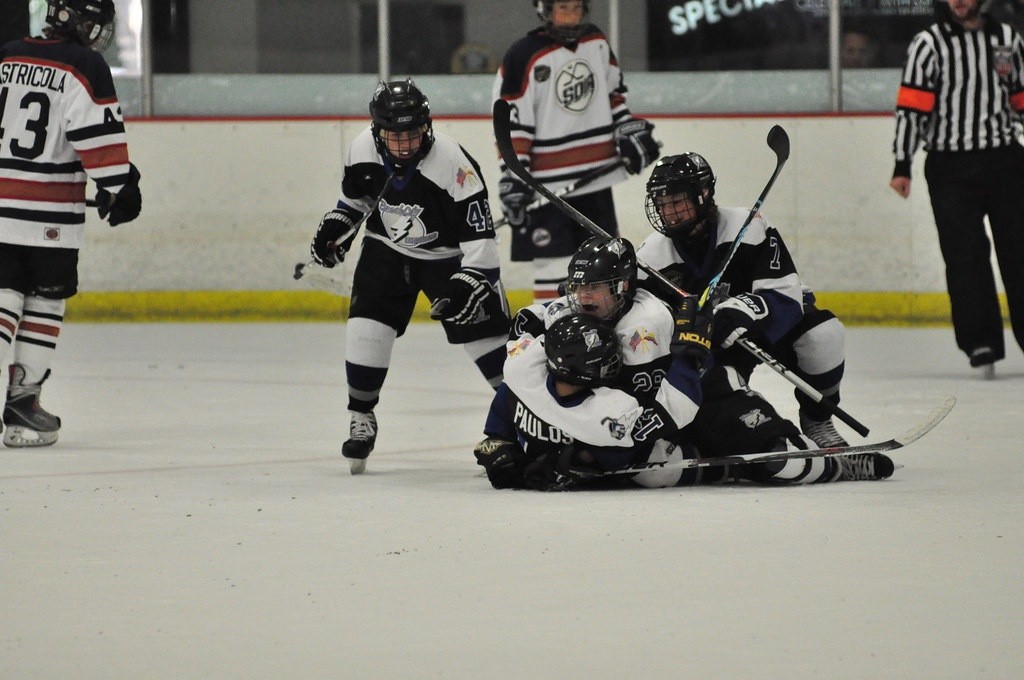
[696,124,792,309]
[331,170,399,245]
[492,97,872,437]
[567,395,956,478]
[492,140,664,227]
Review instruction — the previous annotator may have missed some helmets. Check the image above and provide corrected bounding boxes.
[645,152,716,241]
[545,314,623,388]
[565,234,638,327]
[532,0,591,42]
[45,0,116,54]
[369,77,433,165]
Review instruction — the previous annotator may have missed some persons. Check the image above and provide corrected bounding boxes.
[491,0,662,304]
[890,0,1024,378]
[472,154,895,491]
[310,76,513,475]
[843,28,879,68]
[0,0,143,447]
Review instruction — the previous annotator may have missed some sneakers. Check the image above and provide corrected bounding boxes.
[828,452,905,482]
[341,411,378,474]
[3,364,61,447]
[794,386,849,448]
[970,344,1005,380]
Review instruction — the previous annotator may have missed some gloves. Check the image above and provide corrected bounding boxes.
[670,297,715,372]
[429,269,495,328]
[499,172,537,230]
[96,161,142,226]
[614,119,660,174]
[474,436,526,490]
[713,292,770,350]
[311,208,359,269]
